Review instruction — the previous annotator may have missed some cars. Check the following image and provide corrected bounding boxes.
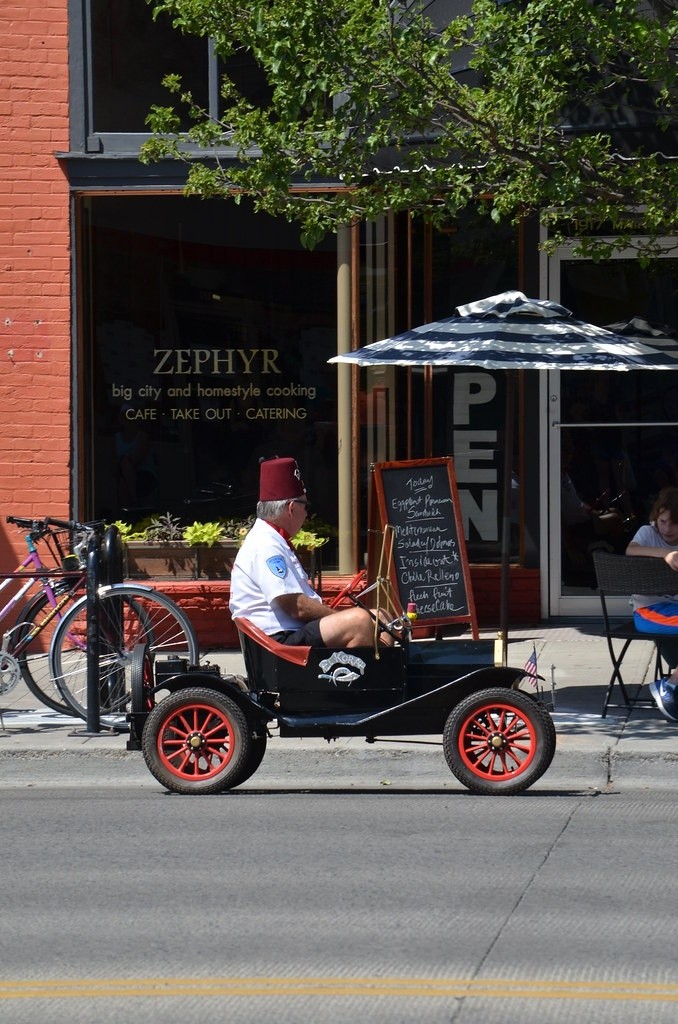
[124,568,557,794]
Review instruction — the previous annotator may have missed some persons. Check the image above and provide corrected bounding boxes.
[229,379,266,432]
[652,427,678,490]
[627,487,678,723]
[568,376,633,510]
[112,421,158,508]
[228,456,395,648]
[278,395,314,427]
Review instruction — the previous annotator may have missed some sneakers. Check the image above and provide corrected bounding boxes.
[649,677,678,720]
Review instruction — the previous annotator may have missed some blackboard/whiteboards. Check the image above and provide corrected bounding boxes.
[367,457,476,624]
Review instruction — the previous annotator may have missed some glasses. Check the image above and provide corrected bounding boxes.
[288,500,310,511]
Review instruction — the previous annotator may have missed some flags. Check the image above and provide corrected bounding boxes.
[524,649,538,688]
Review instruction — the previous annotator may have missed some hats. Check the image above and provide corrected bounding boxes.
[259,457,306,501]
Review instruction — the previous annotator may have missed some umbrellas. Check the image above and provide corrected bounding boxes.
[325,288,678,671]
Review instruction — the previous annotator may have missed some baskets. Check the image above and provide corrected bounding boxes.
[52,519,106,569]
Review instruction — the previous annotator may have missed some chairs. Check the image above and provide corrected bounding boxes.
[232,614,334,700]
[593,550,678,719]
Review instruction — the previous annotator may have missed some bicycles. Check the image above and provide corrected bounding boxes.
[0,514,200,734]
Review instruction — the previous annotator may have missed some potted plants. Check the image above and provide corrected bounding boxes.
[100,510,327,580]
